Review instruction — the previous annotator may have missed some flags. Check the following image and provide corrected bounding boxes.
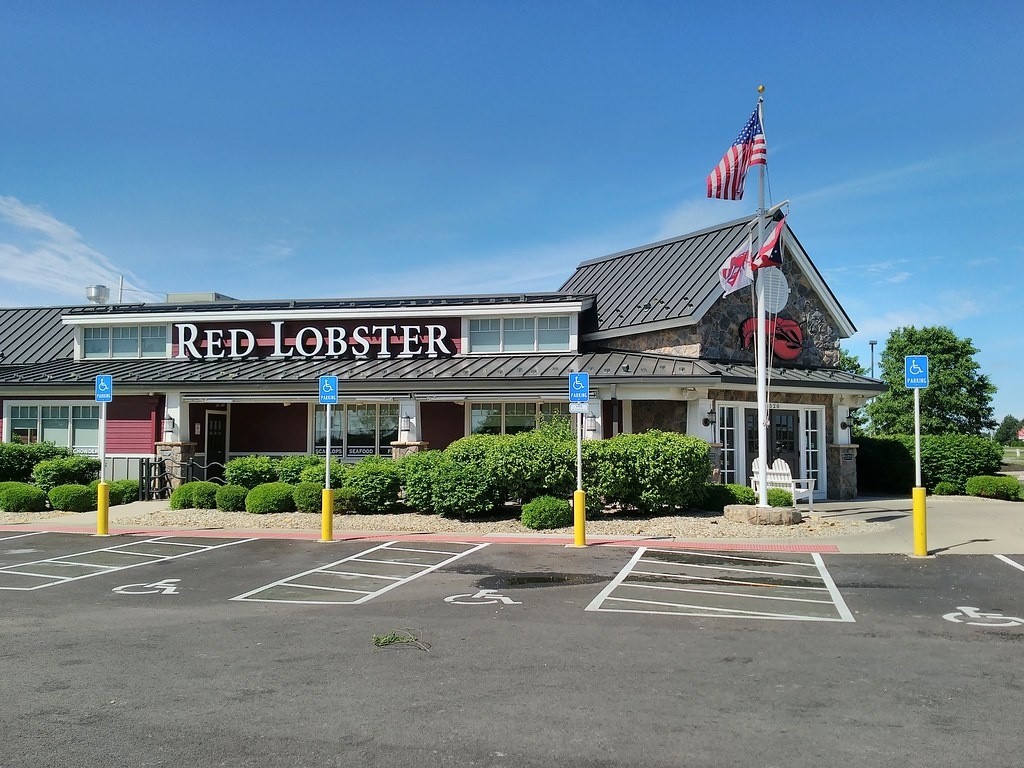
[718,232,754,299]
[705,96,769,201]
[751,213,791,272]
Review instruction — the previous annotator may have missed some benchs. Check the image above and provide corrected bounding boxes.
[750,456,816,510]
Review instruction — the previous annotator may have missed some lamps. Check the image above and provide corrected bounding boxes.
[703,410,716,426]
[163,413,175,432]
[841,413,854,430]
[586,411,596,430]
[400,411,411,431]
